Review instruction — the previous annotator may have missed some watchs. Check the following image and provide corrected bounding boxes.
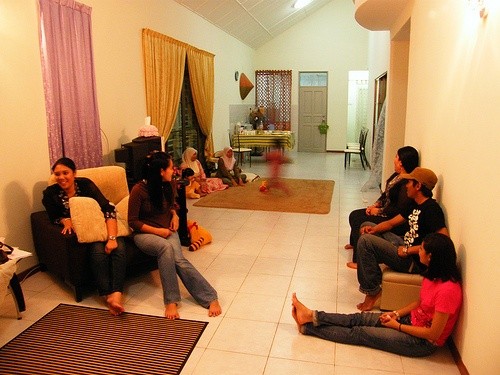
[108,236,117,240]
[402,246,409,255]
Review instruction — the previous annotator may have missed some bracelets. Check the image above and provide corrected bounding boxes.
[393,311,400,321]
[399,323,402,332]
[173,214,179,220]
[63,218,71,223]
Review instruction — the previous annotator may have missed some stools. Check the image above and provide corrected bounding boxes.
[379,263,424,311]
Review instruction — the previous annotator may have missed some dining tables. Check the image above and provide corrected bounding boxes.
[230,130,296,164]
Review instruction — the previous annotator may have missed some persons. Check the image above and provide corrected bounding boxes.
[215,147,247,187]
[180,148,227,193]
[182,168,207,199]
[344,146,418,269]
[127,152,222,320]
[259,137,294,197]
[42,158,125,316]
[357,167,449,311]
[291,233,463,357]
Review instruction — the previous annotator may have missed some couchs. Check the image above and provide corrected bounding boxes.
[30,165,160,303]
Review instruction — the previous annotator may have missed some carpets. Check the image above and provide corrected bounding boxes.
[0,303,210,375]
[192,177,336,215]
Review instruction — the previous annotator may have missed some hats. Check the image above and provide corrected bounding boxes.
[240,72,254,100]
[400,166,438,191]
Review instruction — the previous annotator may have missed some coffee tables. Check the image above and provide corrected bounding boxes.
[0,245,34,321]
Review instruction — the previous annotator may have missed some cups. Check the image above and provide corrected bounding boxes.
[268,124,274,130]
[247,124,253,130]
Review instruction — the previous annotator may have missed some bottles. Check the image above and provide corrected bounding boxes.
[259,121,264,130]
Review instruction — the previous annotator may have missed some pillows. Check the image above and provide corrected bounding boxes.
[68,193,133,244]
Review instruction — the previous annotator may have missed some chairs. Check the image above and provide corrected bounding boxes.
[228,132,252,168]
[343,126,372,172]
